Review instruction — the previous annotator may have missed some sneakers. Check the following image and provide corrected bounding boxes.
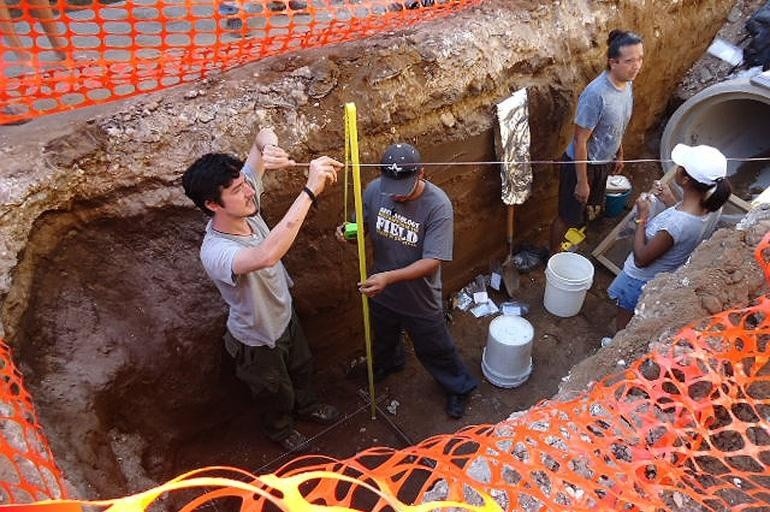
[227,19,249,38]
[447,396,465,417]
[374,360,408,384]
[312,403,339,424]
[601,337,612,347]
[271,0,309,15]
[280,429,311,453]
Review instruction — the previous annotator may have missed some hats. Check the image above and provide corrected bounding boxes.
[379,143,421,197]
[670,143,728,186]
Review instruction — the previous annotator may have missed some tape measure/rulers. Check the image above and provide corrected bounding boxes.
[343,102,376,421]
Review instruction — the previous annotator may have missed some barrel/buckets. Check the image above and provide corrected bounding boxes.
[543,253,595,317]
[604,188,630,214]
[480,315,534,388]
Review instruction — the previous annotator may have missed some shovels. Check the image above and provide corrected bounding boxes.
[564,226,587,245]
[501,205,521,298]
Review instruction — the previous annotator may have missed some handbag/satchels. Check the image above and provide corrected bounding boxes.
[743,1,769,65]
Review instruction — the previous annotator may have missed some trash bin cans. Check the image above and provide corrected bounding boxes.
[605,191,631,217]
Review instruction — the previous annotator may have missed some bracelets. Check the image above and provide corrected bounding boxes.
[302,184,318,203]
[635,218,648,224]
[260,143,276,158]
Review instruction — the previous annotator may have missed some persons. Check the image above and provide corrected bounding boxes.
[182,128,345,453]
[217,0,312,39]
[334,143,479,420]
[541,28,646,262]
[598,142,733,349]
